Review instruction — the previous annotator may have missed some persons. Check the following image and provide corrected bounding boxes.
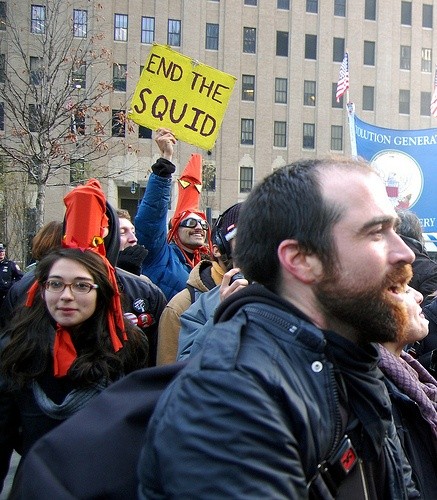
[137,159,420,500]
[0,129,437,500]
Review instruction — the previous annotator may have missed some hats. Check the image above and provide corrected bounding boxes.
[0,243,7,250]
[211,202,242,245]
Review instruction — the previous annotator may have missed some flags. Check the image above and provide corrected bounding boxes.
[430,68,437,119]
[336,53,349,103]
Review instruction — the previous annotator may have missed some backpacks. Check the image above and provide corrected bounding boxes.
[10,362,183,500]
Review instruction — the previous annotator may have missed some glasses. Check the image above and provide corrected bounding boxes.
[43,280,98,294]
[179,218,209,230]
